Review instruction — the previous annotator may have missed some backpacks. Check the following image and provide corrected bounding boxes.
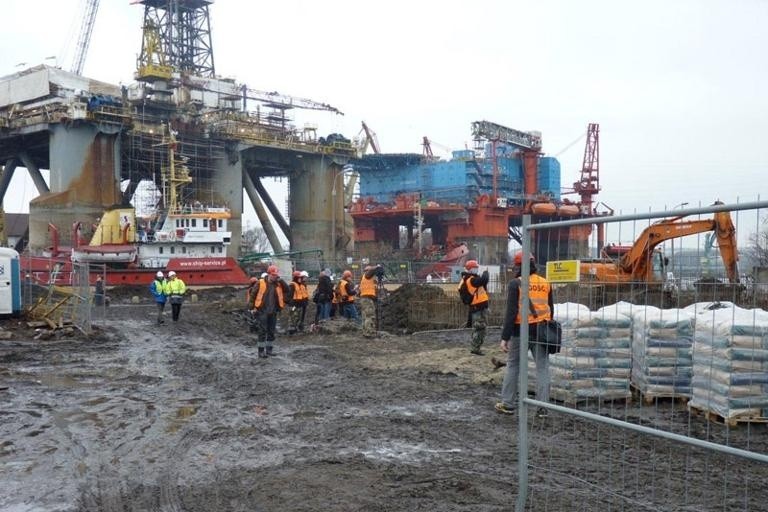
[458,276,478,305]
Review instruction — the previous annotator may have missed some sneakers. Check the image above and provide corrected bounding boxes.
[471,350,486,357]
[535,408,548,418]
[495,401,514,414]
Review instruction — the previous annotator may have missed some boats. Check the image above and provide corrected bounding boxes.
[18,122,258,296]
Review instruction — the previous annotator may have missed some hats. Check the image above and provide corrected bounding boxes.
[156,269,178,277]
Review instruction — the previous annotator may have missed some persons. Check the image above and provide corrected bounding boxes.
[246,260,385,358]
[459,260,490,356]
[166,271,186,321]
[150,272,168,322]
[494,250,555,418]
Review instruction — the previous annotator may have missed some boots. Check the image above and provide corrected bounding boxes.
[258,344,276,356]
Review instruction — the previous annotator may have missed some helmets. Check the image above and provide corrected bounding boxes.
[247,265,380,281]
[514,250,535,262]
[465,258,478,269]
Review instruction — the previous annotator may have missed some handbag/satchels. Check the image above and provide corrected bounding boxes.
[535,317,563,353]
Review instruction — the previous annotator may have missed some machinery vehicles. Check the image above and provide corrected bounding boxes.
[580,200,743,290]
